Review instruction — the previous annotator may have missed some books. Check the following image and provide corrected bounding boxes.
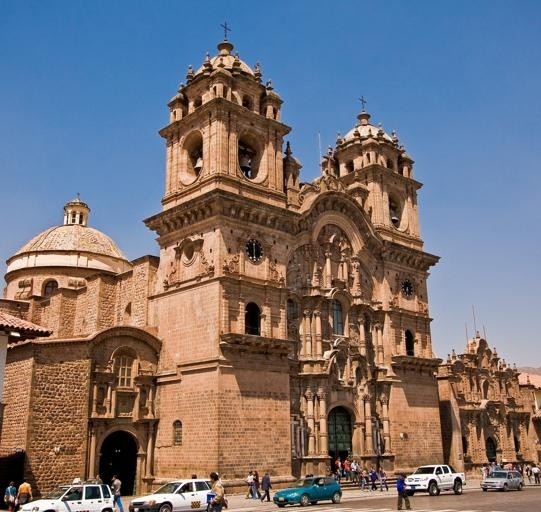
[206,493,216,504]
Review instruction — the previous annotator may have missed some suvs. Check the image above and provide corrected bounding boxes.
[129,479,223,511]
[20,479,115,512]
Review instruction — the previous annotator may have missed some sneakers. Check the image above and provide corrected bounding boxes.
[335,480,389,491]
[245,496,271,502]
[398,507,411,510]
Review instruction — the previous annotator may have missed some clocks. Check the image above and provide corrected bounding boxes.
[246,239,266,265]
[401,278,414,298]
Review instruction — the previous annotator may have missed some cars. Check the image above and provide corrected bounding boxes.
[272,476,344,507]
[480,470,525,491]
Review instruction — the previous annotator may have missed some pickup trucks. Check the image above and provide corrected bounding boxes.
[404,463,467,497]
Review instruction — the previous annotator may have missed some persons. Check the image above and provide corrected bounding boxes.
[205,472,224,512]
[63,488,79,501]
[110,474,124,512]
[3,481,16,512]
[395,471,411,511]
[252,471,261,500]
[332,457,388,493]
[93,474,103,484]
[16,477,33,508]
[260,472,272,503]
[243,470,252,500]
[480,460,541,485]
